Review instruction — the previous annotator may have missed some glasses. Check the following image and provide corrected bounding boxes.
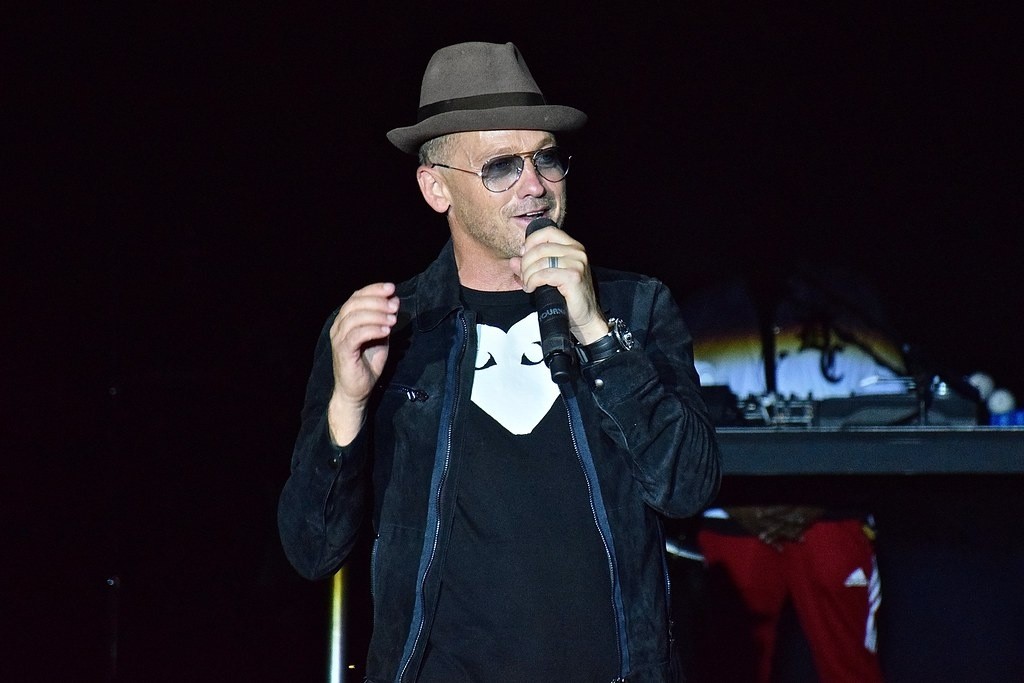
[431,146,572,192]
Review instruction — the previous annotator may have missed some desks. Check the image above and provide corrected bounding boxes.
[716,423,1024,477]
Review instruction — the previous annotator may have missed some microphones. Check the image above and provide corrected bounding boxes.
[526,217,573,383]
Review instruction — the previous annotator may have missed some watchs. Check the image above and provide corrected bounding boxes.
[575,317,636,366]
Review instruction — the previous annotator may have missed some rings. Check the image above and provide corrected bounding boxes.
[549,256,558,268]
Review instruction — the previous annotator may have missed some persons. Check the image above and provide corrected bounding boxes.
[278,45,724,683]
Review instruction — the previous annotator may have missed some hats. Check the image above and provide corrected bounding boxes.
[386,42,588,153]
